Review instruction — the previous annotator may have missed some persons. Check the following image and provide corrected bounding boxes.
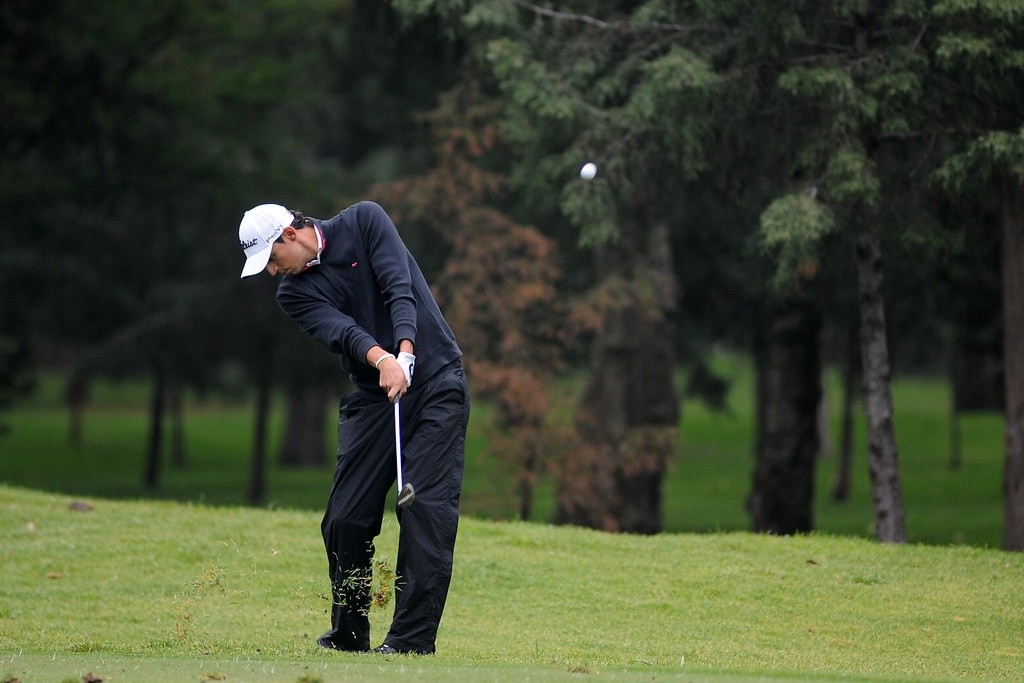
[238,200,471,656]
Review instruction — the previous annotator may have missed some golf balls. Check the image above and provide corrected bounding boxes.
[579,163,597,180]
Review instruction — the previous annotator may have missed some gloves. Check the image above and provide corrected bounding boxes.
[396,351,416,387]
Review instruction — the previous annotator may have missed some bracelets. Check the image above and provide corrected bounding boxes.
[375,353,395,369]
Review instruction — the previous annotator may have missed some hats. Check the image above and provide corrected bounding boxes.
[238,204,295,280]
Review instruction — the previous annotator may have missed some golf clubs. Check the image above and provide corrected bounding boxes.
[393,389,414,508]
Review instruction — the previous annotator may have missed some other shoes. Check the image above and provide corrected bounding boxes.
[372,643,436,653]
